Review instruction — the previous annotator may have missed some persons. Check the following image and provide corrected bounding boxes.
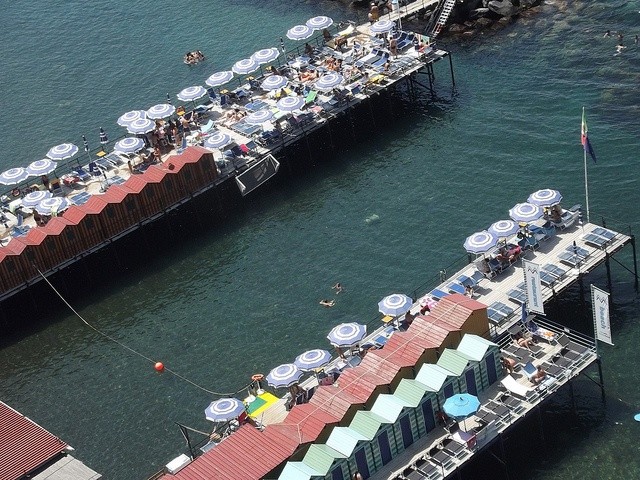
[271,66,278,74]
[218,109,246,126]
[635,35,639,45]
[390,37,398,59]
[618,35,623,43]
[614,43,627,57]
[530,365,545,386]
[41,175,50,189]
[406,310,414,327]
[604,30,610,38]
[152,118,191,147]
[332,282,343,294]
[513,332,538,351]
[384,60,390,71]
[297,69,318,82]
[304,43,312,53]
[418,41,429,51]
[182,49,205,64]
[361,72,369,94]
[440,268,447,284]
[549,211,561,222]
[371,3,379,21]
[419,39,424,48]
[333,346,346,359]
[500,356,512,376]
[325,55,342,71]
[127,161,133,173]
[554,205,562,213]
[465,285,473,298]
[319,298,336,308]
[153,147,161,164]
[368,10,373,23]
[251,133,268,147]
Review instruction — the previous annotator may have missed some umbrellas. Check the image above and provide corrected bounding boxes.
[21,190,53,208]
[0,167,28,198]
[113,137,146,154]
[442,393,481,434]
[99,127,109,153]
[46,143,79,178]
[231,58,260,75]
[176,86,207,108]
[205,398,246,423]
[315,74,345,91]
[82,135,92,164]
[35,197,70,216]
[250,49,280,64]
[488,220,520,244]
[306,16,333,30]
[245,110,274,124]
[205,71,234,95]
[378,293,413,329]
[117,110,147,127]
[527,189,563,207]
[509,202,544,225]
[294,349,333,382]
[266,363,304,398]
[370,20,396,33]
[463,230,499,259]
[146,104,176,120]
[280,37,287,62]
[126,119,156,134]
[327,321,367,357]
[206,133,231,149]
[167,92,172,104]
[286,25,314,40]
[276,97,306,111]
[26,159,58,176]
[260,75,288,91]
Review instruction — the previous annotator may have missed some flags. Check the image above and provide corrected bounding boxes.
[581,106,598,162]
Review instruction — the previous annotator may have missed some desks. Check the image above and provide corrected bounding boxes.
[382,316,393,327]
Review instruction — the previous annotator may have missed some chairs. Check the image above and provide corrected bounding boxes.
[71,192,93,206]
[475,261,496,280]
[538,362,564,377]
[542,263,565,277]
[547,354,572,368]
[371,74,388,84]
[62,173,78,186]
[507,323,544,357]
[539,271,556,288]
[556,334,588,354]
[365,50,383,65]
[93,157,112,169]
[355,61,365,72]
[566,245,589,257]
[517,282,524,290]
[206,37,325,108]
[506,288,526,304]
[415,456,438,477]
[437,434,465,455]
[497,390,521,408]
[343,69,362,82]
[523,320,558,344]
[325,46,354,65]
[379,66,398,77]
[359,48,379,62]
[373,52,390,68]
[373,334,387,345]
[491,252,511,273]
[431,288,449,299]
[592,227,616,240]
[51,180,63,195]
[110,175,125,185]
[499,246,517,265]
[73,165,91,181]
[392,56,415,70]
[340,356,361,372]
[491,301,512,317]
[501,375,531,398]
[475,409,499,424]
[447,282,465,294]
[443,420,473,445]
[231,87,360,158]
[105,153,121,164]
[428,444,451,466]
[396,33,414,50]
[497,334,532,367]
[403,465,424,480]
[558,251,583,266]
[519,205,573,250]
[286,395,302,410]
[360,342,384,349]
[295,387,314,400]
[522,362,550,383]
[382,31,401,47]
[582,233,607,248]
[457,275,479,291]
[10,225,30,239]
[483,399,509,417]
[387,32,408,47]
[556,345,580,361]
[487,309,504,323]
[500,350,527,374]
[387,326,400,335]
